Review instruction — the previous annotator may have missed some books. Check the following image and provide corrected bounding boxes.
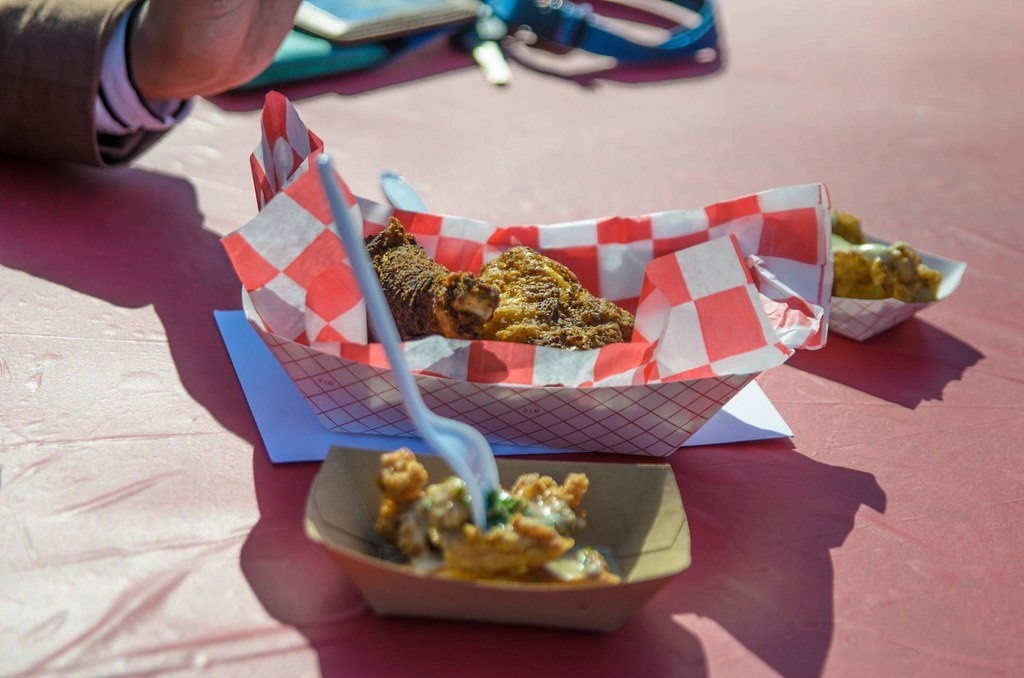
[293,0,486,42]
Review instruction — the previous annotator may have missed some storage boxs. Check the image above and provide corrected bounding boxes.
[241,284,763,456]
[304,445,693,630]
[829,231,967,340]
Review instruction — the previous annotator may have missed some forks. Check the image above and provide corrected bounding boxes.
[315,152,501,527]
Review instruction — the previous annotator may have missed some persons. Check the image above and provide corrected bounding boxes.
[0,0,303,169]
